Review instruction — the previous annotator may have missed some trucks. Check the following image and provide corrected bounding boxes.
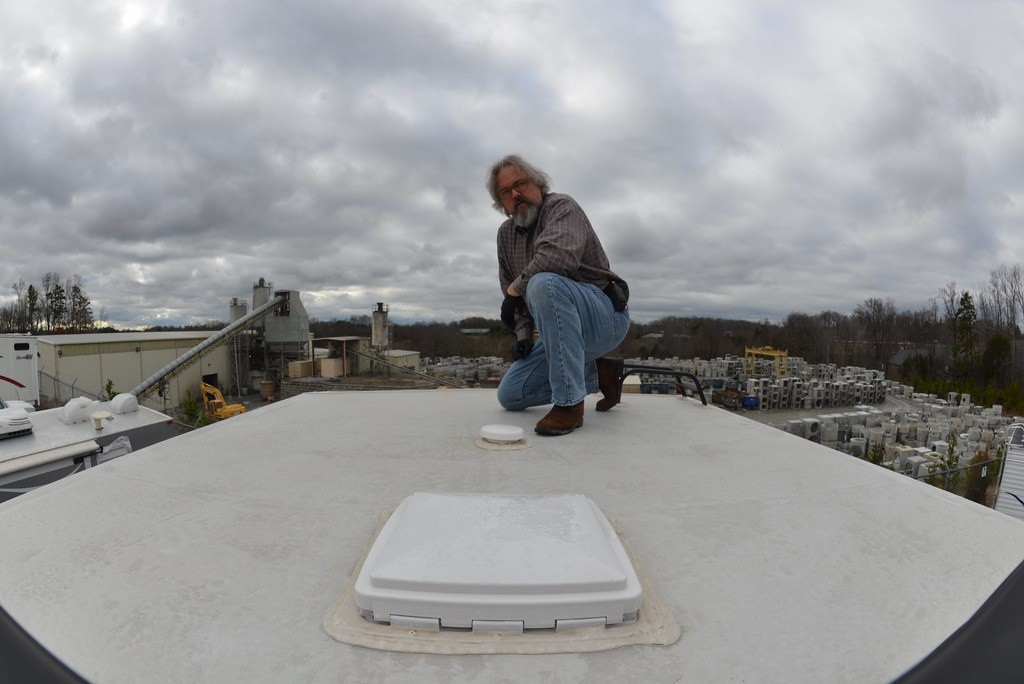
[711,388,758,411]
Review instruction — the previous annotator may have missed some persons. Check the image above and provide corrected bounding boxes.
[486,154,630,436]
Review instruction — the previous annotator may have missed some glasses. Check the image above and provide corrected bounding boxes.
[498,179,530,200]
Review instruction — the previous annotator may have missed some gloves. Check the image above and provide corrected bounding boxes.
[512,339,534,361]
[501,293,525,330]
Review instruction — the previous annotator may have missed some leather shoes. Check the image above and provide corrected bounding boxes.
[535,400,584,435]
[595,357,624,412]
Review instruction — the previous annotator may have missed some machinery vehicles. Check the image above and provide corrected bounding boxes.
[0,374,173,502]
[200,382,246,419]
